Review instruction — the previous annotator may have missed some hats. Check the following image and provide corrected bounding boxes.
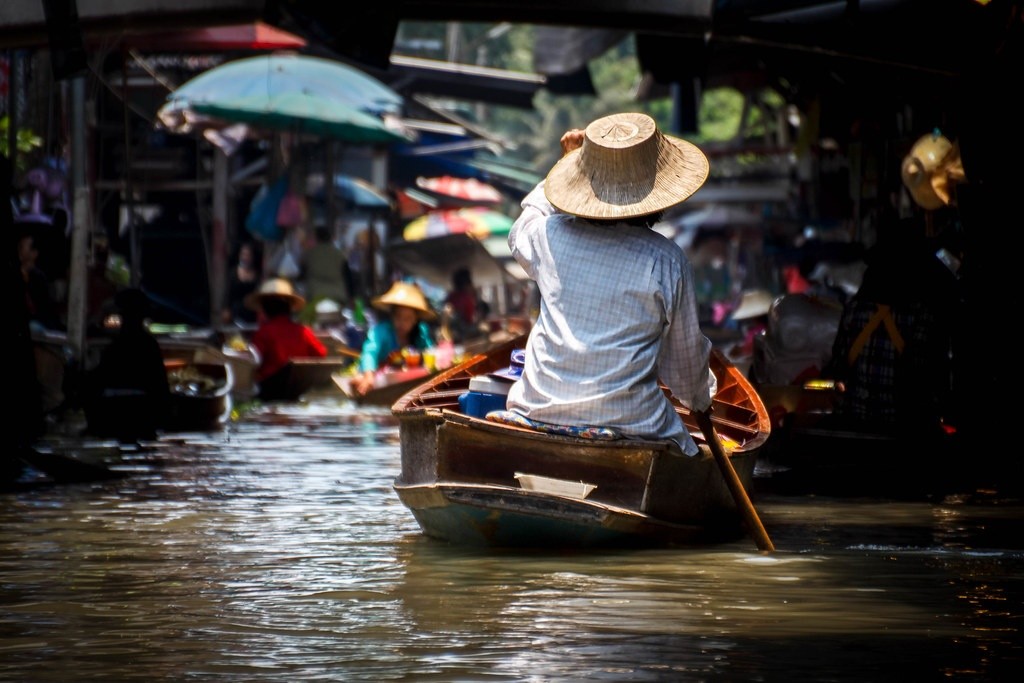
[545,111,711,220]
[372,280,436,320]
[244,280,307,313]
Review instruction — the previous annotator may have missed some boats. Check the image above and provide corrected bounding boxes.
[723,335,966,504]
[32,315,539,479]
[391,334,771,553]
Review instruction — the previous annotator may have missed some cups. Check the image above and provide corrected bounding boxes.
[388,344,467,375]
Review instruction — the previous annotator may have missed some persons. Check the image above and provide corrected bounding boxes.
[87,283,171,395]
[730,291,776,356]
[507,109,722,461]
[236,222,487,398]
[828,218,958,439]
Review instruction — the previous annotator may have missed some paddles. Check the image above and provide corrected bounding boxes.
[695,414,777,552]
